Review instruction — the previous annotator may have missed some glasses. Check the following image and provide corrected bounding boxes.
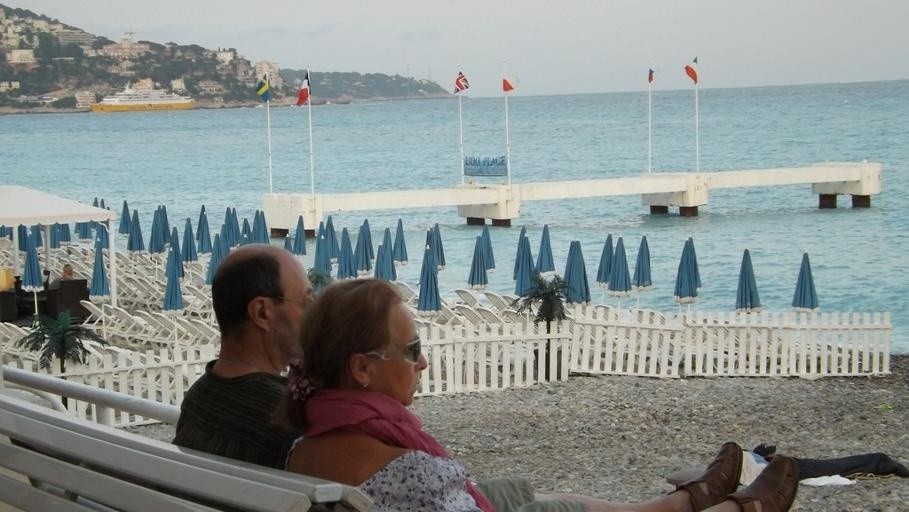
[360,335,422,364]
[265,293,317,310]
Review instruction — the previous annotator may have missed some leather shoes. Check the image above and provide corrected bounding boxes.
[668,440,743,512]
[720,453,798,512]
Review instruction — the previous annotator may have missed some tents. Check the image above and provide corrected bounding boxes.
[0,182,118,309]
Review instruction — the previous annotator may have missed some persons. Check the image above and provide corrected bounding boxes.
[173,244,316,467]
[283,279,800,512]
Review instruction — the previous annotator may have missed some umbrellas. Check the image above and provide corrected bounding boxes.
[468,220,654,305]
[688,236,704,290]
[792,251,819,312]
[738,247,762,312]
[674,240,698,312]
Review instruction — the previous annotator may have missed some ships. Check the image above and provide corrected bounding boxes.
[91,79,198,113]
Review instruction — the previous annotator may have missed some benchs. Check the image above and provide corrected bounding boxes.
[1,400,375,512]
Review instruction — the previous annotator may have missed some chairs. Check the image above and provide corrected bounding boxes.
[0,245,222,397]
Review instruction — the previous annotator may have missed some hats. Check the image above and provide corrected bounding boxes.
[63,264,73,272]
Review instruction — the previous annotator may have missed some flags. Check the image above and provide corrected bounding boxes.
[255,69,273,102]
[645,68,655,89]
[685,58,701,86]
[455,68,468,91]
[502,66,519,96]
[296,72,310,107]
